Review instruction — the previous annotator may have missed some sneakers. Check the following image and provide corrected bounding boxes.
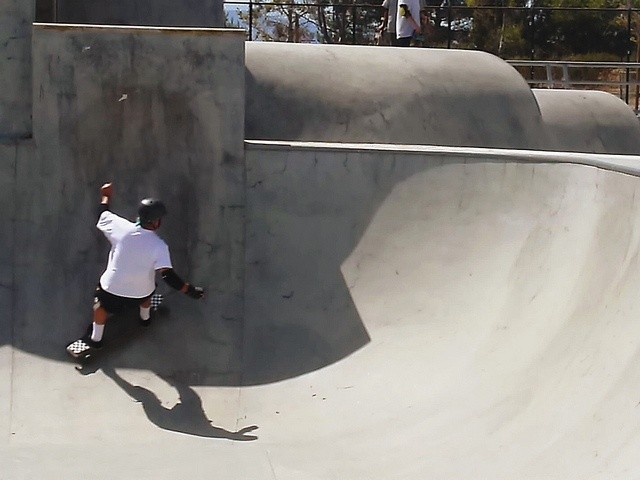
[139,316,152,327]
[82,334,104,348]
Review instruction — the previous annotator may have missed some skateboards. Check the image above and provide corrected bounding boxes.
[65,291,164,362]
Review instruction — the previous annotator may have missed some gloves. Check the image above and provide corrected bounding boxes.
[185,286,203,299]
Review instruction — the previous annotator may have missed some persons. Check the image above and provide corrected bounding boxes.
[379,0,395,45]
[395,0,423,47]
[83,183,205,351]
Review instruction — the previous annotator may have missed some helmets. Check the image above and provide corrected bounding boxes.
[138,198,168,221]
